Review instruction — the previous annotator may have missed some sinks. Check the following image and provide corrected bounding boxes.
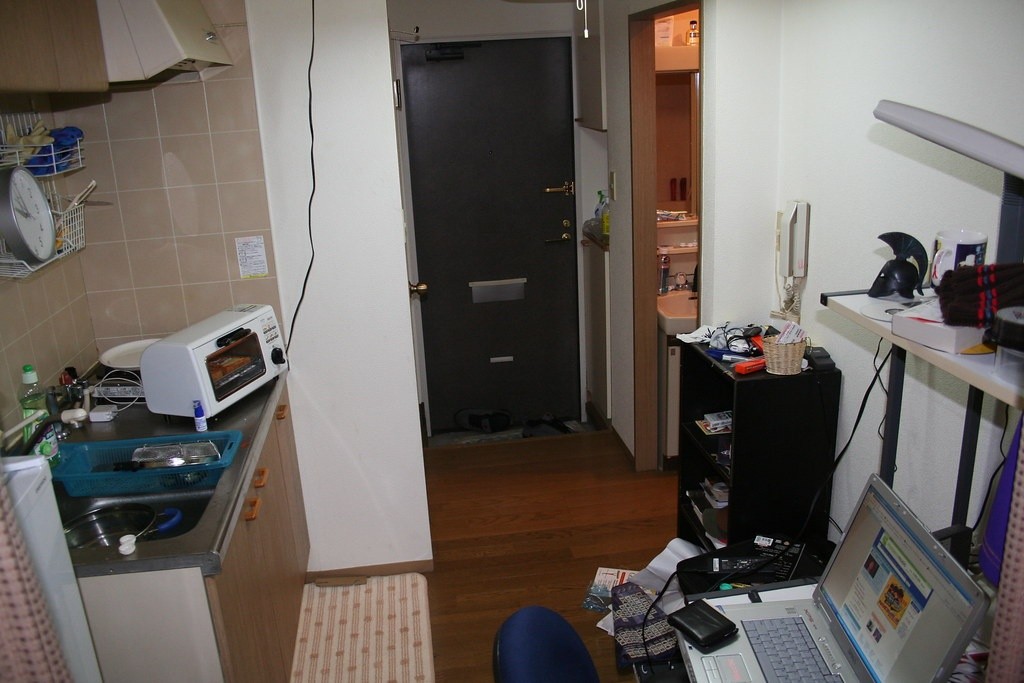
[63,490,215,550]
[657,291,697,335]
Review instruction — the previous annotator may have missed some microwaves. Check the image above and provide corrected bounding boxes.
[140,304,289,419]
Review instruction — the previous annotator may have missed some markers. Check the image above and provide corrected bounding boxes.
[706,348,752,362]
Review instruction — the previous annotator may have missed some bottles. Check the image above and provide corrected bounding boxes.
[595,190,611,234]
[193,401,208,432]
[18,365,61,468]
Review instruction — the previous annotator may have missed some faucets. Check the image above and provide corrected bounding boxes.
[21,408,88,455]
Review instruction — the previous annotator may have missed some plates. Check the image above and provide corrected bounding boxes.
[99,339,169,371]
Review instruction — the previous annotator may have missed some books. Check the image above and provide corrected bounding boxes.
[695,411,733,435]
[698,475,728,509]
[891,297,985,355]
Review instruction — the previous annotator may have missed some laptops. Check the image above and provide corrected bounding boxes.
[673,473,991,683]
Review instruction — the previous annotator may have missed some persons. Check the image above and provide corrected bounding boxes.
[865,561,874,575]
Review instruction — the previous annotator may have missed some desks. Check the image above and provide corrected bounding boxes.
[675,287,1024,683]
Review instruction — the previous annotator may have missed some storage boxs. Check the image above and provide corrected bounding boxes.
[590,567,640,605]
[704,410,733,428]
[891,296,986,355]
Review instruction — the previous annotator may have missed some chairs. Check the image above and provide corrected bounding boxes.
[492,606,600,683]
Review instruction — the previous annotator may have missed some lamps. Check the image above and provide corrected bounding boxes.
[873,100,1024,265]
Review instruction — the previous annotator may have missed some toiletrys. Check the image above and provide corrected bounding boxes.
[655,15,674,47]
[656,211,687,221]
[686,20,699,47]
[657,255,671,296]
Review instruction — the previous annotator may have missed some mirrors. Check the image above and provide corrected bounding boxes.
[654,72,696,220]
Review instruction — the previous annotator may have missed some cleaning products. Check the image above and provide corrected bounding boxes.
[594,190,609,235]
[17,364,62,470]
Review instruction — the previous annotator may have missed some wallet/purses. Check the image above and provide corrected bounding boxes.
[667,599,739,650]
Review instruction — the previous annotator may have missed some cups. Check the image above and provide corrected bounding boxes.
[929,229,988,295]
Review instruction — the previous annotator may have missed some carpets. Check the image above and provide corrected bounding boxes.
[289,572,436,683]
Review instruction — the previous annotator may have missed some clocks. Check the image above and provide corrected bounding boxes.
[0,167,56,266]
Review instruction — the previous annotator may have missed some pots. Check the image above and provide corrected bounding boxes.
[55,502,182,548]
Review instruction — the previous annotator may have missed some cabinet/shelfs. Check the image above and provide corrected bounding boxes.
[572,0,674,132]
[678,329,843,560]
[657,218,698,254]
[78,379,310,683]
[580,234,612,433]
[0,111,86,280]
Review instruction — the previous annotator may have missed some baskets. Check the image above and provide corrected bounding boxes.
[52,430,242,497]
[762,336,807,376]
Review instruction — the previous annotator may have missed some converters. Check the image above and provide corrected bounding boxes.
[707,555,776,579]
